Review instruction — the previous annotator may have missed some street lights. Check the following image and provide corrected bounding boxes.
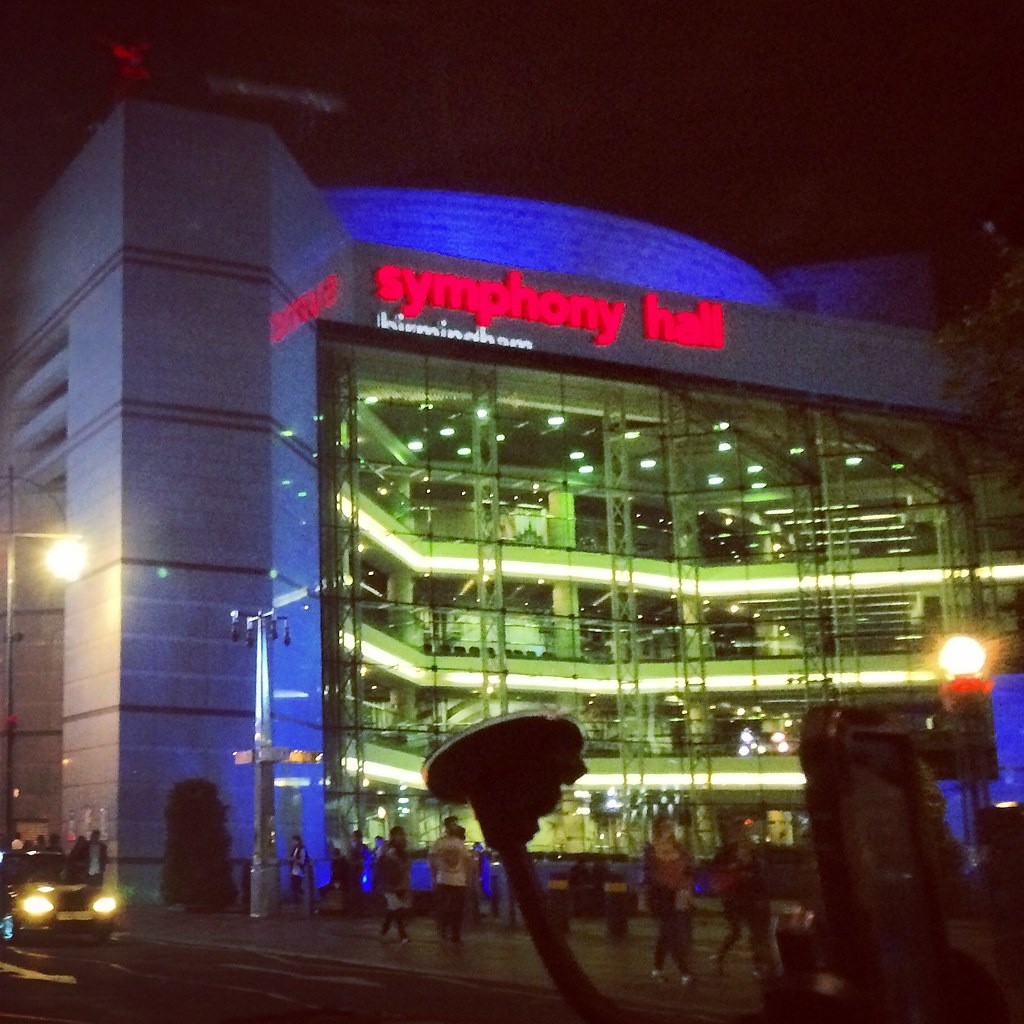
[938,634,992,876]
[0,466,91,853]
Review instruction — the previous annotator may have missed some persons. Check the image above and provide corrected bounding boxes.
[709,813,776,983]
[287,835,306,902]
[427,816,465,946]
[369,826,419,943]
[328,830,365,920]
[641,814,695,989]
[0,823,67,884]
[65,830,108,885]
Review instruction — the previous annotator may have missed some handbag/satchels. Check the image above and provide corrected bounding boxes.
[676,891,697,914]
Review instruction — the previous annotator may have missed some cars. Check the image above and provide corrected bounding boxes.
[0,860,117,945]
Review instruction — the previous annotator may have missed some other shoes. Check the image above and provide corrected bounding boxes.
[402,938,411,944]
[651,970,666,982]
[681,975,691,986]
